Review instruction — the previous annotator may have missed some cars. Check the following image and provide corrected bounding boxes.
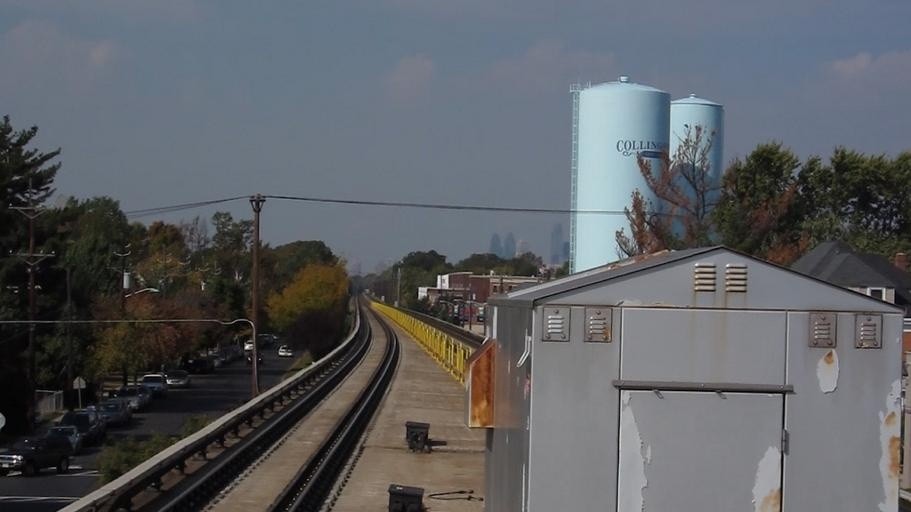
[277,344,295,358]
[0,334,275,478]
